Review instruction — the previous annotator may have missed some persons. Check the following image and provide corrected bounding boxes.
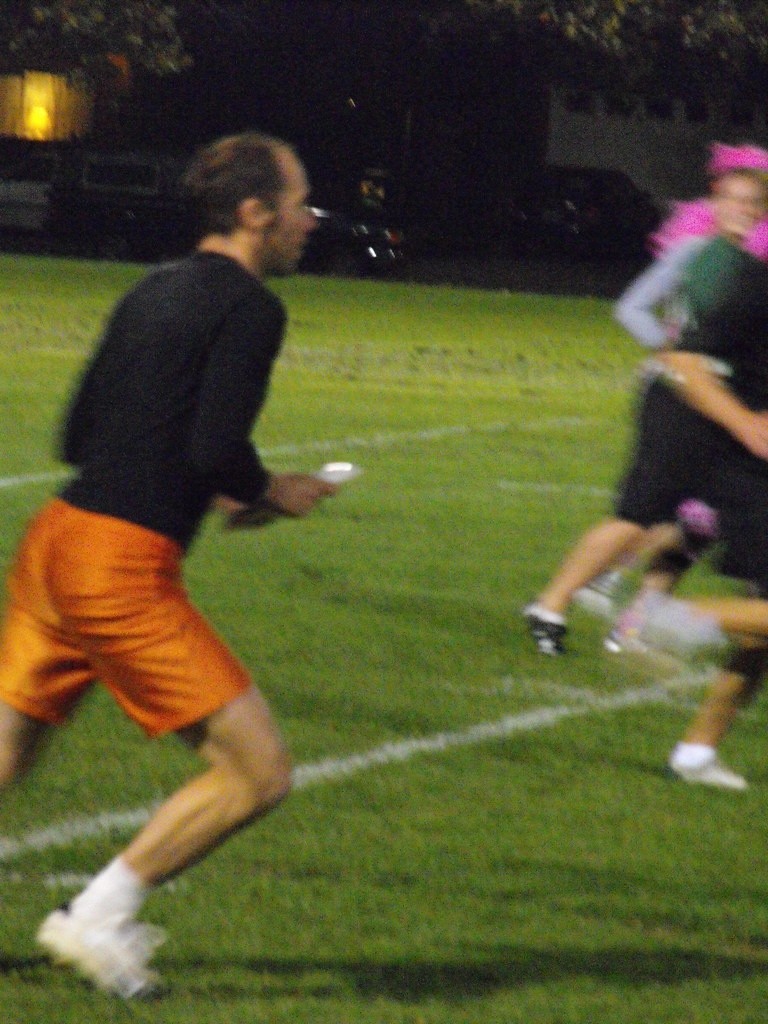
[0,133,334,1002]
[524,143,768,790]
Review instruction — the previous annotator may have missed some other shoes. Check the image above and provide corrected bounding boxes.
[35,900,170,999]
[604,632,652,656]
[523,612,568,657]
[667,754,746,791]
